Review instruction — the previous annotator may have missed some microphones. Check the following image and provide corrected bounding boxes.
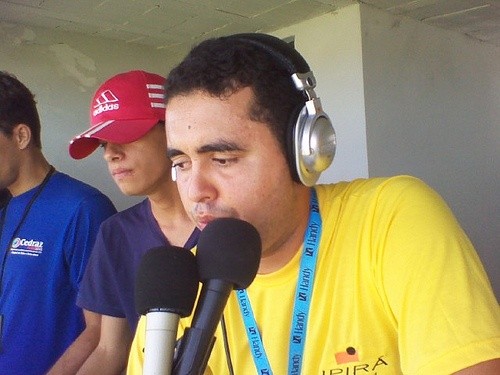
[137,245,199,375]
[171,217,262,375]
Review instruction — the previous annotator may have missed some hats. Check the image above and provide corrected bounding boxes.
[69,70,168,160]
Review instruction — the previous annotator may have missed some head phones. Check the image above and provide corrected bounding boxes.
[172,33,337,188]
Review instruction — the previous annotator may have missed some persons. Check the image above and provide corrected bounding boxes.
[75,69,202,375]
[126,34,500,375]
[0,70,119,375]
